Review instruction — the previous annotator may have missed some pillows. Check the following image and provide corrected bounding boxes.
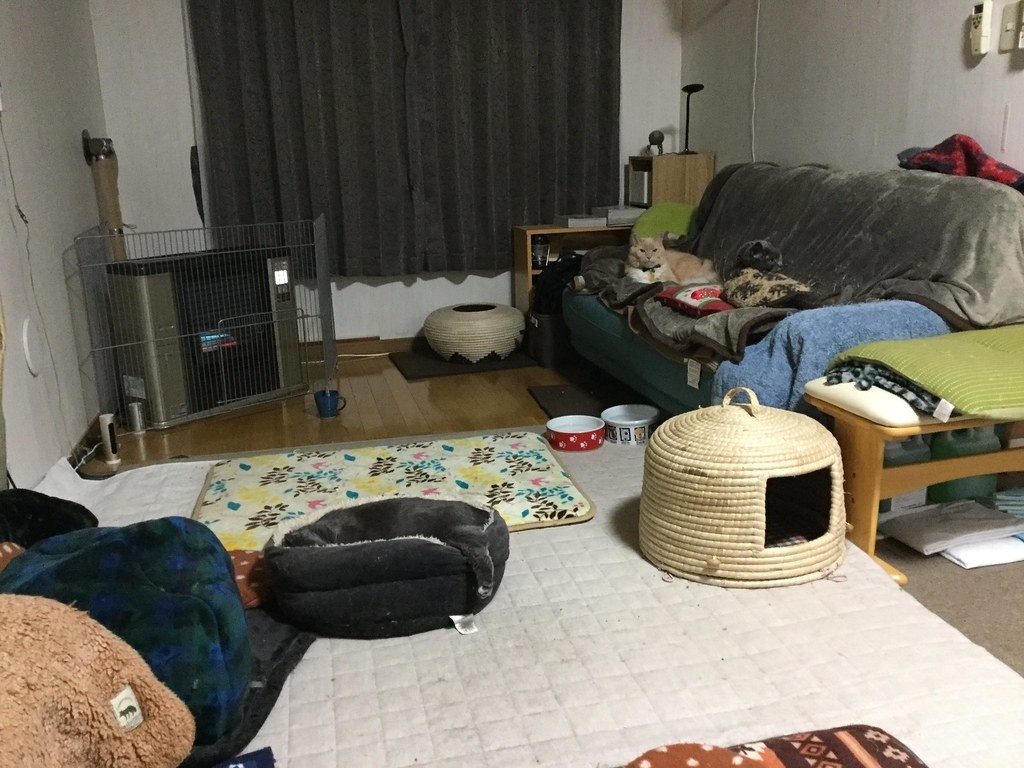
[723,267,812,308]
[0,592,196,768]
[654,282,739,318]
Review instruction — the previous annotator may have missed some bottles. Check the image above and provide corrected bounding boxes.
[933,425,1002,504]
[878,434,932,514]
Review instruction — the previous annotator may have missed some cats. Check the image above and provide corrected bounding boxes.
[623,229,721,286]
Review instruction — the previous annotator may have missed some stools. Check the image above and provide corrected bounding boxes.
[803,354,1024,585]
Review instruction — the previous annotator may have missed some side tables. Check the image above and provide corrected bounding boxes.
[510,152,716,314]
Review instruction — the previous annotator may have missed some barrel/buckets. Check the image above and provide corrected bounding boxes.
[526,304,565,360]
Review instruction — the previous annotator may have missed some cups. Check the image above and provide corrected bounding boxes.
[314,390,346,419]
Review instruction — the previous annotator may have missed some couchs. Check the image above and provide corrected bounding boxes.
[558,161,1024,510]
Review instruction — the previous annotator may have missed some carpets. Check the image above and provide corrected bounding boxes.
[32,424,1023,768]
[189,430,597,555]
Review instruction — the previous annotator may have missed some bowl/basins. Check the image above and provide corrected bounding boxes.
[545,415,605,453]
[601,404,660,445]
